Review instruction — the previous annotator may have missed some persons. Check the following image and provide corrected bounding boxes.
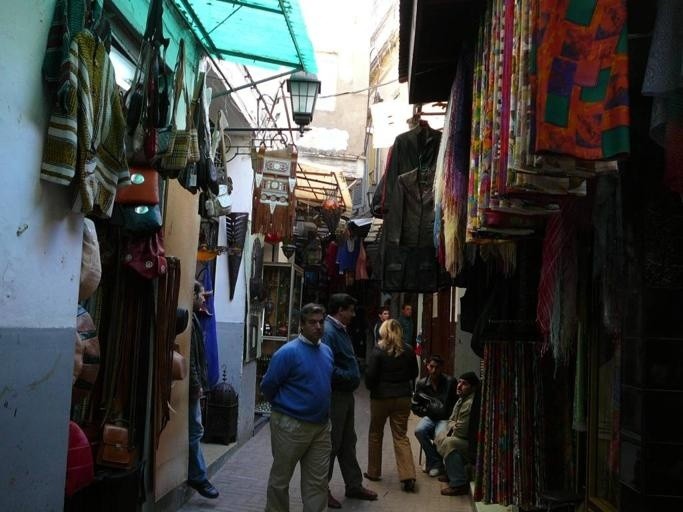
[361,318,419,493]
[410,356,457,477]
[321,293,381,508]
[431,369,480,496]
[259,299,337,511]
[397,303,413,346]
[189,277,219,498]
[372,306,391,345]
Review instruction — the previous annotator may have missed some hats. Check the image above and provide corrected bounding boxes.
[175,307,189,335]
[460,372,480,386]
[70,301,100,407]
[169,342,187,389]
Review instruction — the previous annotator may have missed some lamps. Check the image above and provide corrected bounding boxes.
[213,69,322,137]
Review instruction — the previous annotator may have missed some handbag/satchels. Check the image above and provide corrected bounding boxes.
[119,228,169,281]
[114,167,159,204]
[123,37,219,195]
[118,205,164,238]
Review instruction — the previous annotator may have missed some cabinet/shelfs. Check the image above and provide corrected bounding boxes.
[262,265,305,342]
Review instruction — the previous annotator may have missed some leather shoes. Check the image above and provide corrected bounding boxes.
[345,486,378,500]
[327,494,342,508]
[428,468,469,496]
[404,480,415,491]
[364,472,378,481]
[188,479,219,498]
